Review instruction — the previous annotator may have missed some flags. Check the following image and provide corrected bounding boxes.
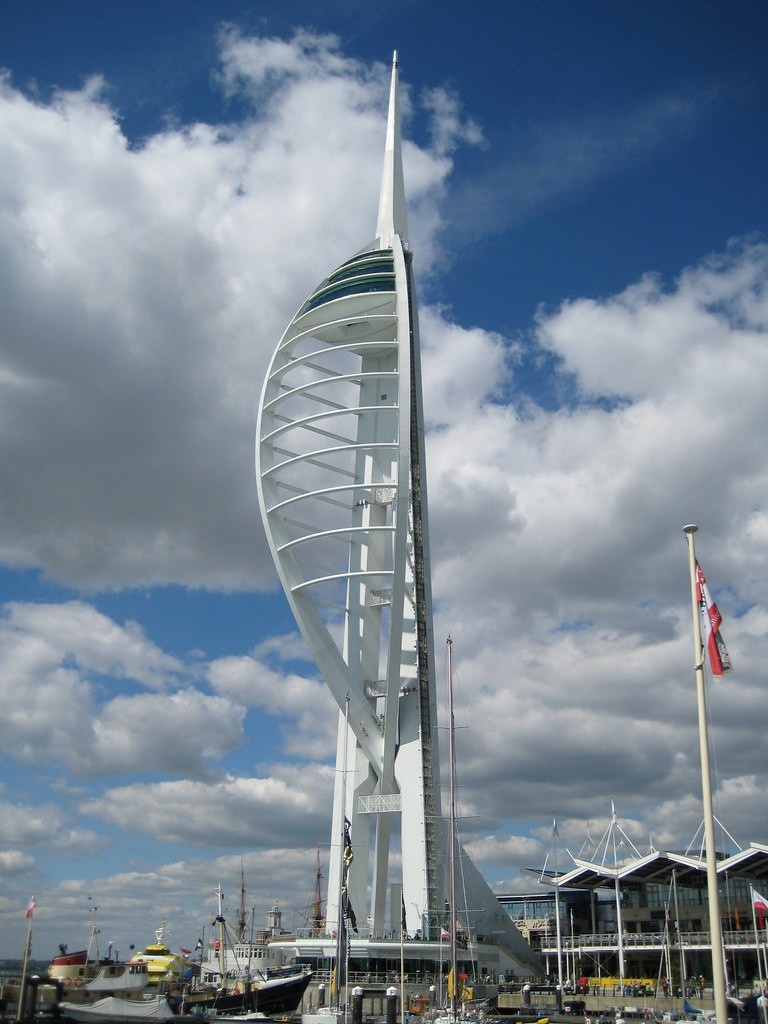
[194,938,203,950]
[441,927,451,939]
[26,896,36,918]
[753,889,768,913]
[695,561,734,683]
[402,896,407,933]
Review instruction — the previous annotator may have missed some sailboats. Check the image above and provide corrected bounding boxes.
[421,629,502,1024]
[652,870,734,1024]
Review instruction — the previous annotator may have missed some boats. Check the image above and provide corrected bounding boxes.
[0,848,327,1024]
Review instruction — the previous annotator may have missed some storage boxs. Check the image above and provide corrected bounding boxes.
[625,1006,640,1013]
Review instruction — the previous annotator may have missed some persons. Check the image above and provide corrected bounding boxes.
[625,975,705,999]
[477,975,589,995]
